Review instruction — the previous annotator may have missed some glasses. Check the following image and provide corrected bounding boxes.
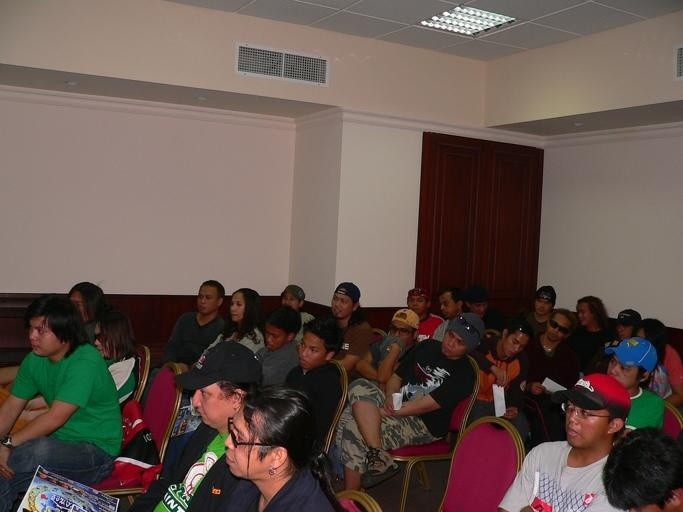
[456,314,481,338]
[226,416,271,449]
[565,403,609,418]
[93,333,103,341]
[519,326,532,335]
[393,328,411,333]
[548,318,569,335]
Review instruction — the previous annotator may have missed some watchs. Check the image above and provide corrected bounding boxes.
[0,435,15,451]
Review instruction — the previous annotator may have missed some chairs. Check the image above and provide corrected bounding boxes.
[484,326,503,342]
[86,362,183,497]
[334,489,382,512]
[367,327,390,345]
[316,359,348,457]
[383,353,481,512]
[436,415,525,512]
[658,398,683,443]
[124,342,152,405]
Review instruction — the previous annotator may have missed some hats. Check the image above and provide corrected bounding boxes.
[536,285,556,303]
[603,337,658,372]
[408,288,431,299]
[552,373,631,419]
[280,285,306,300]
[393,309,420,329]
[618,309,640,326]
[335,281,360,302]
[449,312,485,352]
[174,341,263,389]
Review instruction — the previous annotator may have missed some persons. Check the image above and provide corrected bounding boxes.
[432,288,466,341]
[125,340,263,512]
[603,337,664,434]
[284,317,344,444]
[348,309,420,387]
[336,312,486,491]
[189,288,265,372]
[526,285,556,336]
[468,321,534,444]
[602,427,683,512]
[613,309,641,340]
[253,304,300,404]
[573,295,612,374]
[165,280,226,365]
[465,287,507,332]
[67,282,105,324]
[0,292,123,512]
[638,318,683,408]
[406,288,445,341]
[280,284,315,347]
[225,387,344,512]
[330,281,372,371]
[510,308,582,441]
[89,307,138,414]
[495,373,631,512]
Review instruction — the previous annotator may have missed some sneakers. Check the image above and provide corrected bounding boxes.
[361,447,400,487]
